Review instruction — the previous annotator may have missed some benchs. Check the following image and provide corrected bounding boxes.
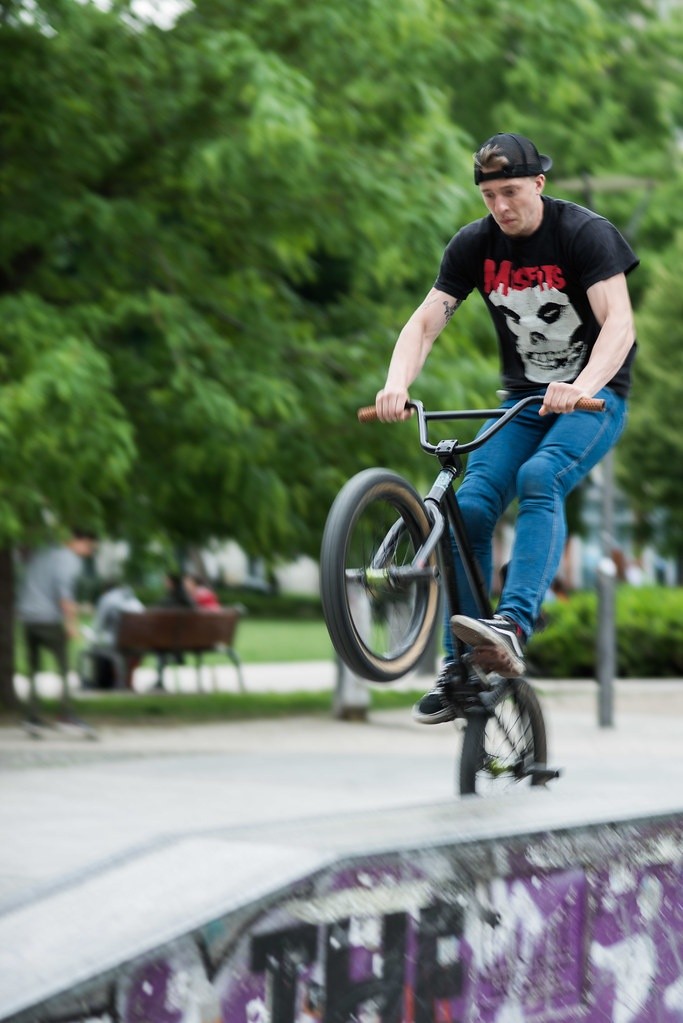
[78,601,251,694]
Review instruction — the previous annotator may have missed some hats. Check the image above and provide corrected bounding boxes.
[474,131,553,185]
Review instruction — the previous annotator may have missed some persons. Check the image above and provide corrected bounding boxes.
[153,575,221,691]
[89,575,146,650]
[21,523,100,683]
[374,134,640,725]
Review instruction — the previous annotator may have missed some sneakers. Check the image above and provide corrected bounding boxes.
[412,659,491,724]
[450,610,528,680]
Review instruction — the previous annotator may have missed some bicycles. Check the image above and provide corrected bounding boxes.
[312,398,607,796]
